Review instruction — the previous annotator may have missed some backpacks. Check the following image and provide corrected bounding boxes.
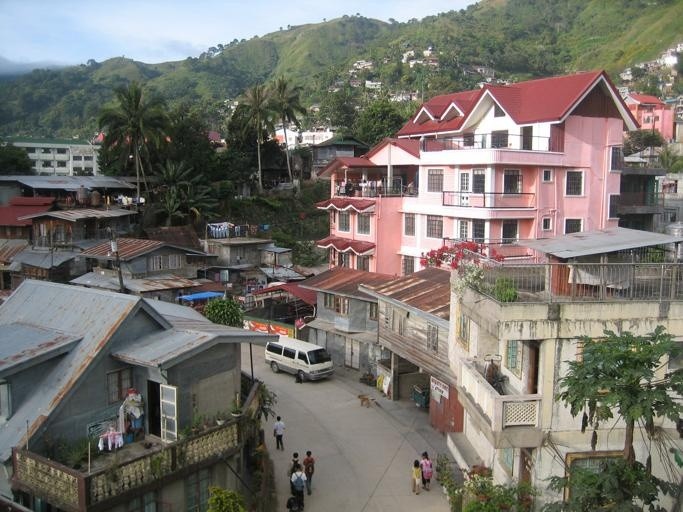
[294,471,305,492]
[422,459,433,480]
[305,458,314,477]
[292,459,299,474]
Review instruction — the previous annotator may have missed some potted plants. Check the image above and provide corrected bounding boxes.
[177,431,187,440]
[103,459,124,489]
[216,417,225,425]
[231,408,243,417]
[192,415,202,437]
[436,452,542,512]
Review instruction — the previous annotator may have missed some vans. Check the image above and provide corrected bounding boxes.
[265,336,335,382]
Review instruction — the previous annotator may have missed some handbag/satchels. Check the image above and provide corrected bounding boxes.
[415,478,421,486]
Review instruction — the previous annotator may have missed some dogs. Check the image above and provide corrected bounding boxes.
[358,393,375,407]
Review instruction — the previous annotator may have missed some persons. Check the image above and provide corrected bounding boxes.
[302,450,315,495]
[344,178,353,197]
[290,462,307,509]
[361,179,368,197]
[419,450,433,490]
[411,459,422,495]
[285,488,302,512]
[273,415,286,451]
[287,452,303,495]
